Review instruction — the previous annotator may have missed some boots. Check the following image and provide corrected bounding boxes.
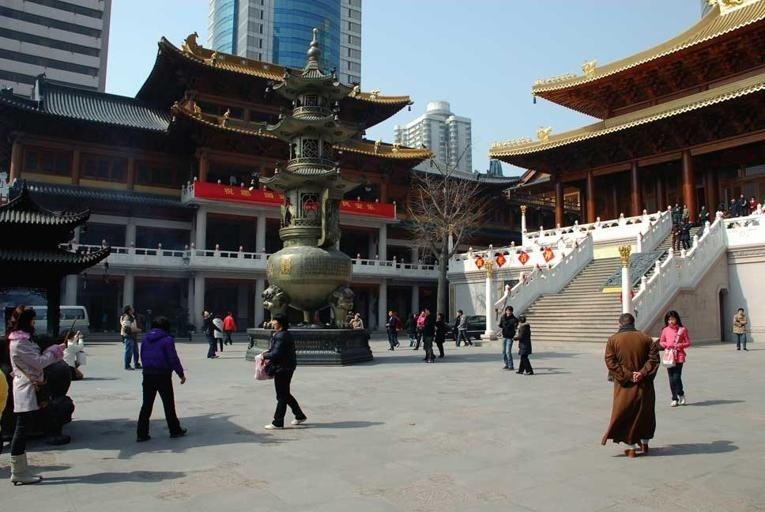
[9,452,43,486]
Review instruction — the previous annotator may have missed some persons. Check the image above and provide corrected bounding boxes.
[262,314,308,430]
[0,300,86,485]
[136,316,187,441]
[201,310,223,358]
[733,307,748,351]
[659,311,690,406]
[667,194,759,250]
[119,305,143,371]
[221,310,236,345]
[500,306,520,369]
[515,316,534,375]
[601,313,662,457]
[212,314,225,352]
[387,306,473,361]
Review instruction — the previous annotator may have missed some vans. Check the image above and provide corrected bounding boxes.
[0,305,90,339]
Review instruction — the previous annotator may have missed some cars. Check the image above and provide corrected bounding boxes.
[446,314,485,339]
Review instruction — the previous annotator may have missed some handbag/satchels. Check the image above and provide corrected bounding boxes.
[254,354,274,382]
[32,376,52,409]
[662,349,678,369]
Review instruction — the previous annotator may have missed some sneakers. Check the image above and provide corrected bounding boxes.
[386,340,474,364]
[291,417,307,425]
[125,365,135,370]
[678,395,685,406]
[136,434,152,442]
[263,424,285,431]
[671,400,679,407]
[637,439,649,453]
[516,372,523,374]
[624,448,637,458]
[205,340,233,359]
[503,366,515,371]
[524,371,534,375]
[736,347,748,351]
[134,363,143,369]
[169,428,187,438]
